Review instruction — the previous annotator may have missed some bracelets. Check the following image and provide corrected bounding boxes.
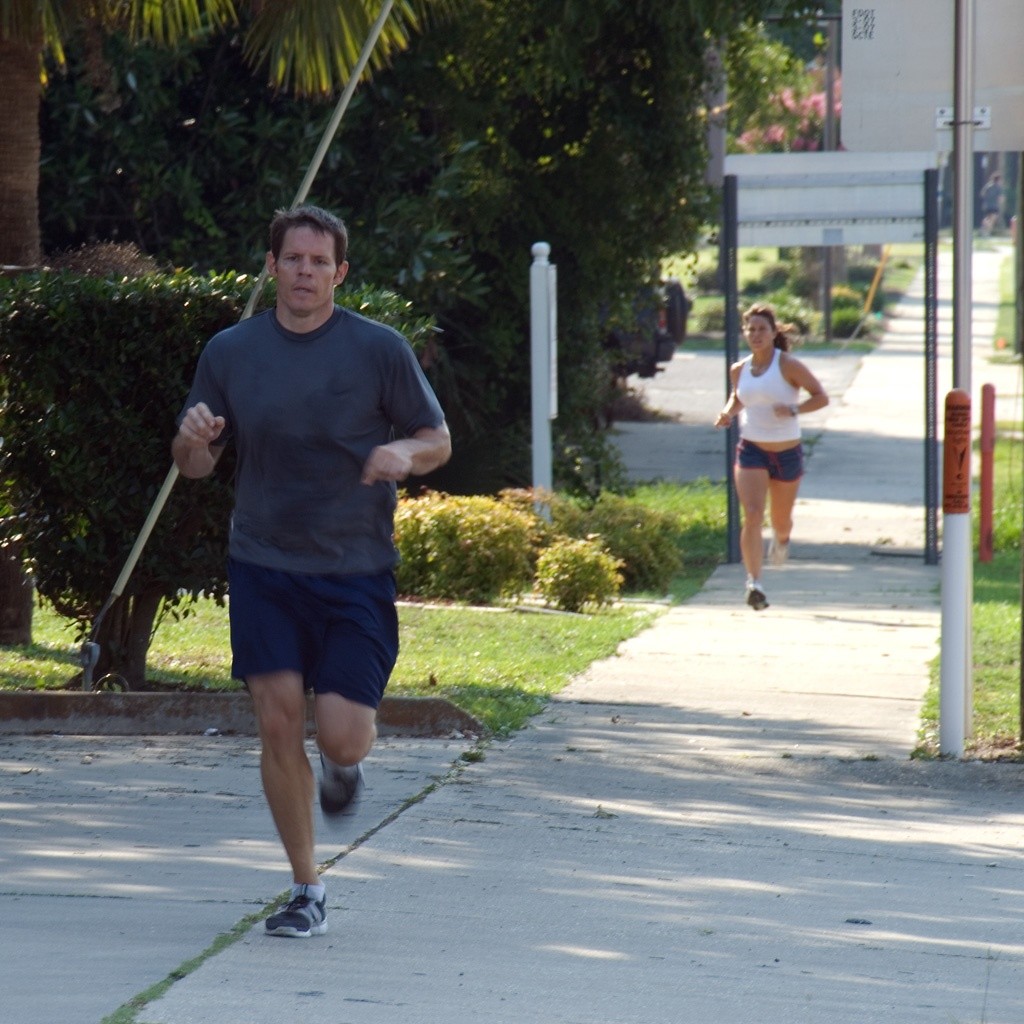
[790,402,798,417]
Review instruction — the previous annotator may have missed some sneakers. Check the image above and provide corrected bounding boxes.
[746,586,770,610]
[265,881,330,939]
[769,536,790,567]
[319,744,364,820]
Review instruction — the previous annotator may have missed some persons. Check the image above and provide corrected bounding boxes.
[169,203,450,937]
[713,303,830,607]
[980,169,1007,240]
